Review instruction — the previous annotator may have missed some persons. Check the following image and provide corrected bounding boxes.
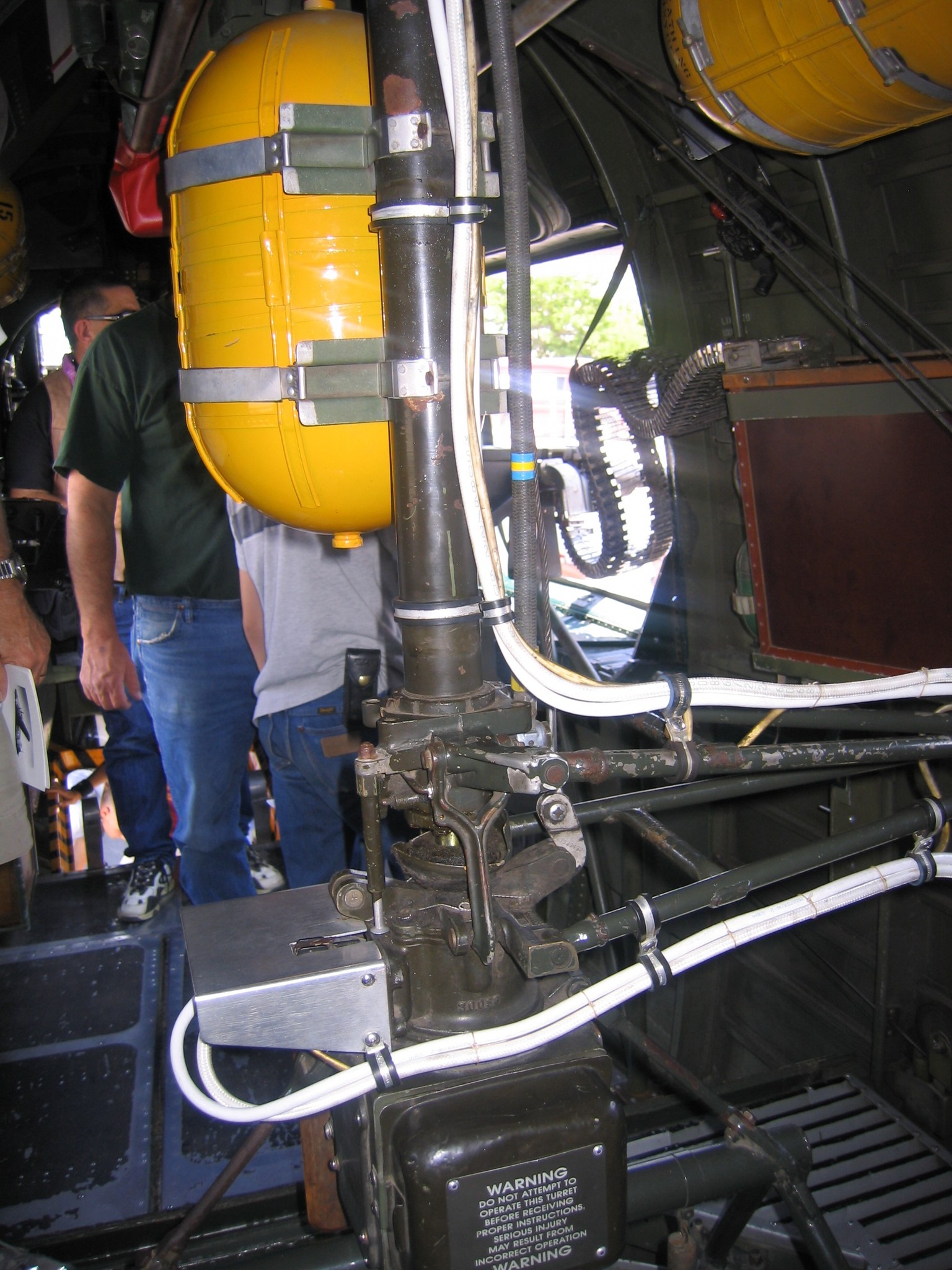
[6,270,286,922]
[223,497,383,885]
[50,294,272,1043]
[1,507,52,926]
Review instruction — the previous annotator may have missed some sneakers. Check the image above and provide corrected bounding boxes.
[245,845,285,895]
[116,855,177,922]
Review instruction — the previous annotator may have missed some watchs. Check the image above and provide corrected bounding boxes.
[1,550,28,585]
